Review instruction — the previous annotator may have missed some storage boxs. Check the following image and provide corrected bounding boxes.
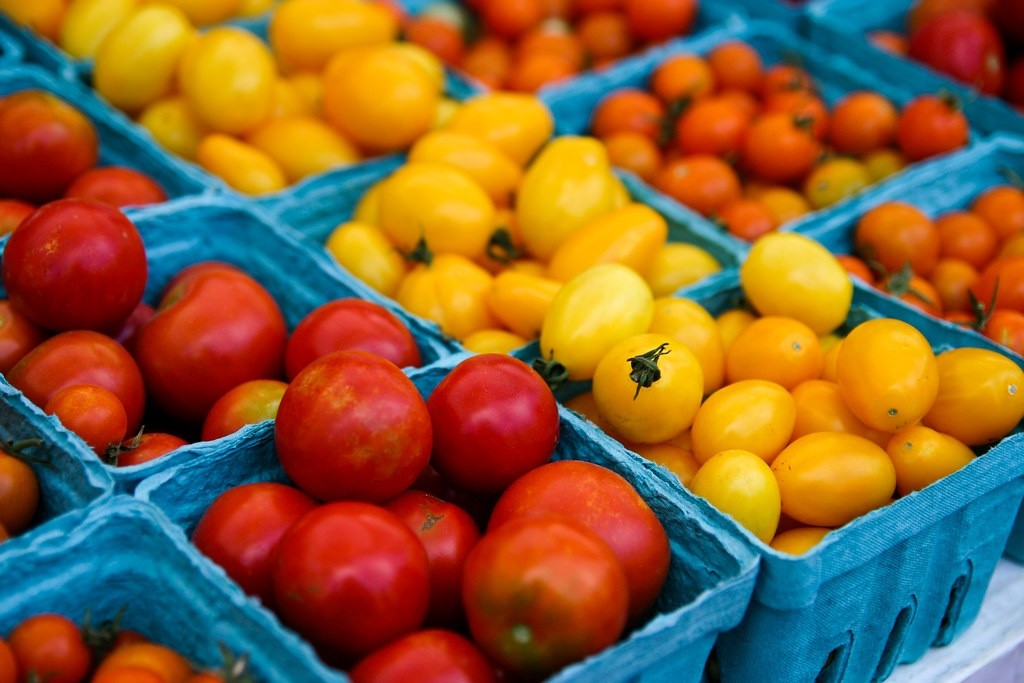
[0,0,1024,683]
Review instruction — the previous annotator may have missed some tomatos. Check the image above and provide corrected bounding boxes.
[0,0,1024,683]
